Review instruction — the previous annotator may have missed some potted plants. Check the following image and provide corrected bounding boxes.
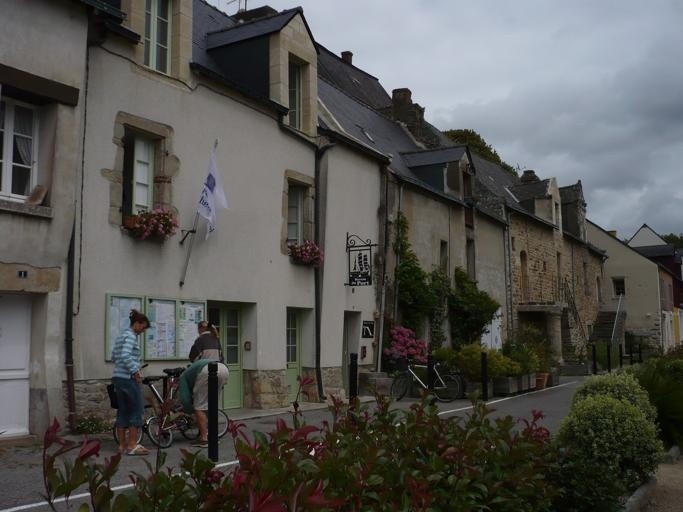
[436,320,561,400]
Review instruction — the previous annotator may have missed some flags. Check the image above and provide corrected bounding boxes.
[205,147,230,211]
[195,185,216,241]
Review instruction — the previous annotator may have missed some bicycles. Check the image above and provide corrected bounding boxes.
[389,359,459,402]
[113,363,228,448]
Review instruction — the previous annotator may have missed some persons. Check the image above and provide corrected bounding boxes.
[187,319,222,364]
[176,360,230,448]
[109,309,154,455]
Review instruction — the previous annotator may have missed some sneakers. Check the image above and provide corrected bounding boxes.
[190,440,208,449]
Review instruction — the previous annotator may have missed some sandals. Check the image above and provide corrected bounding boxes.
[117,444,150,456]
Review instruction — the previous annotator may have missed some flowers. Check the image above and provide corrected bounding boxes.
[285,239,325,267]
[131,200,180,242]
[384,325,429,367]
